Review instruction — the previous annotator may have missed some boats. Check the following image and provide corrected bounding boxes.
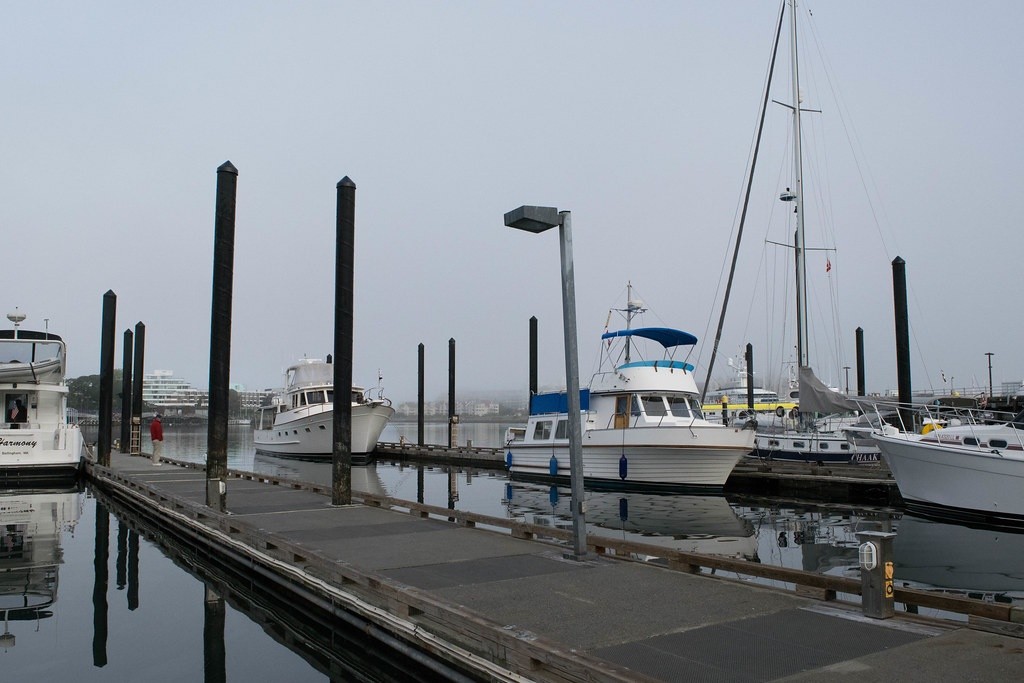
[885,511,1022,601]
[253,351,394,466]
[0,491,89,654]
[0,305,88,489]
[846,398,1024,520]
[504,482,761,566]
[503,280,756,496]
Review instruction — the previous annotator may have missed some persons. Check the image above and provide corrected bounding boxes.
[150,414,163,466]
[979,392,987,413]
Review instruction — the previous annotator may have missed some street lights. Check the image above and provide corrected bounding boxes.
[504,205,599,562]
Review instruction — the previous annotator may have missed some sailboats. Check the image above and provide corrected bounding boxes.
[698,0,899,489]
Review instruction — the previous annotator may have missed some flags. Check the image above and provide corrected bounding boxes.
[11,401,19,419]
